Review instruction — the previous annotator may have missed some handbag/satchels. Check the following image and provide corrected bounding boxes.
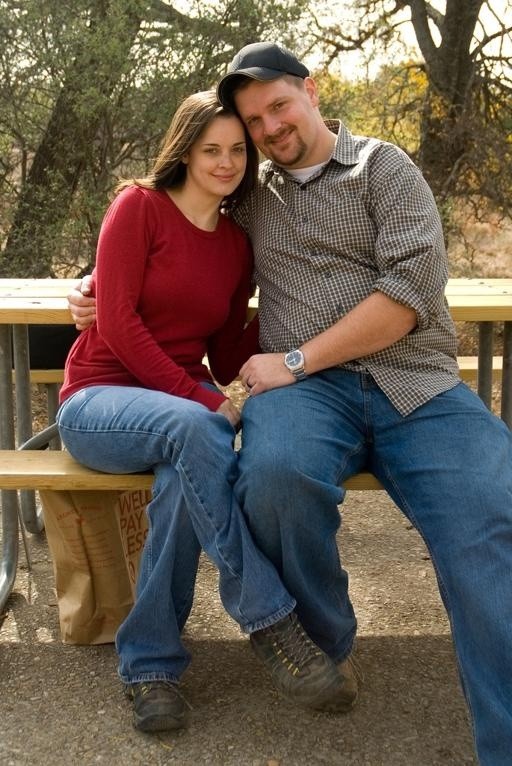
[39,489,152,646]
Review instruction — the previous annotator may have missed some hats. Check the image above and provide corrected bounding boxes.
[216,42,309,115]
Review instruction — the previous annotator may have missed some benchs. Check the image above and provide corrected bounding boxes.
[457,353,504,389]
[0,447,387,609]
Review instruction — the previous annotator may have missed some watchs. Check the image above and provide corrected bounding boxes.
[284,347,307,381]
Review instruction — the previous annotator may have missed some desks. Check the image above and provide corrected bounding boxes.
[0,276,512,609]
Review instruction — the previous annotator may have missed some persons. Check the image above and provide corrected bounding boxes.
[57,91,358,733]
[67,41,512,766]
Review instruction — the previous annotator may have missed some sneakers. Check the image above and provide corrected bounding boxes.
[121,680,189,730]
[249,608,344,708]
[324,650,365,713]
